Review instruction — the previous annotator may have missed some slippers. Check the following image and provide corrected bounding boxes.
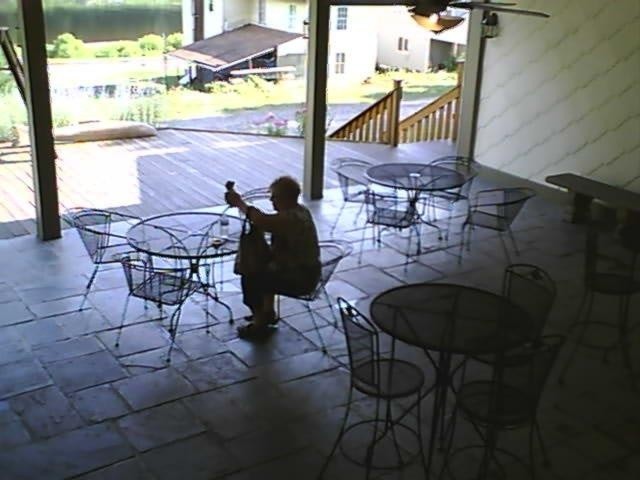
[236,314,280,339]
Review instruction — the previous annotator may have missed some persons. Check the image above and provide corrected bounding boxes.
[220,173,325,342]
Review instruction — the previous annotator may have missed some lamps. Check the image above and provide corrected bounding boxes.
[409,14,464,35]
[480,12,500,39]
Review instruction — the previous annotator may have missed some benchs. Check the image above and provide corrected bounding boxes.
[545,173,640,231]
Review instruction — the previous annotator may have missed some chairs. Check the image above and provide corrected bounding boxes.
[438,262,560,463]
[438,334,567,480]
[560,220,638,387]
[115,256,210,363]
[327,154,539,273]
[316,297,430,480]
[210,187,277,293]
[58,207,155,311]
[269,237,353,356]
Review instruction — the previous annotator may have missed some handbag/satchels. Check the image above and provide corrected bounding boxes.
[233,214,271,276]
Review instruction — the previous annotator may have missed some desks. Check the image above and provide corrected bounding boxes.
[126,211,253,332]
[367,282,536,480]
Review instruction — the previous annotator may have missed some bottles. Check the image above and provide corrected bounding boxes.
[219,214,230,237]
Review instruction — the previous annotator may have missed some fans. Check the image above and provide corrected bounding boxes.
[327,0,549,22]
